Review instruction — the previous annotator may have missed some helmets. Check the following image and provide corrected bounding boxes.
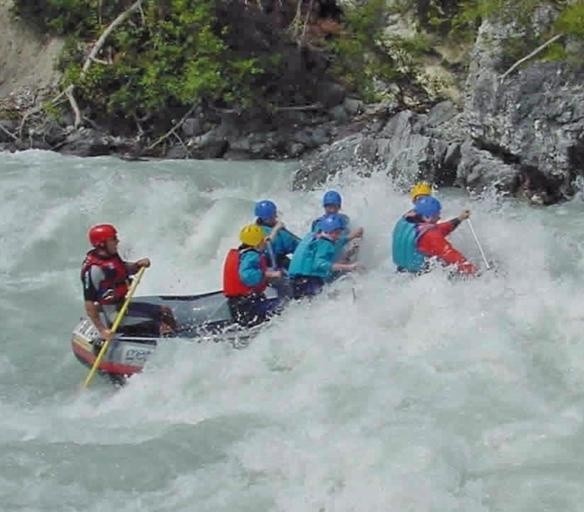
[321,213,344,233]
[240,224,264,246]
[416,196,440,217]
[255,201,275,217]
[89,225,116,245]
[323,192,341,207]
[410,184,432,200]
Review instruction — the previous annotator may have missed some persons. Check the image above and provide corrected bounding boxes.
[311,190,353,236]
[252,200,302,269]
[392,196,479,280]
[288,214,365,299]
[409,184,432,206]
[227,224,285,327]
[80,224,178,340]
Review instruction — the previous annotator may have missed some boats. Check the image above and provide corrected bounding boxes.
[72,290,259,387]
